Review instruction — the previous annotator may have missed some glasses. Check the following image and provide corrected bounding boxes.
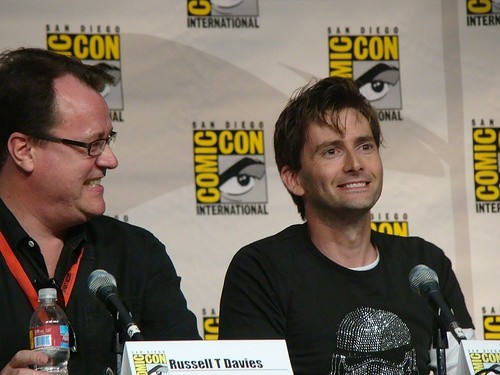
[19,130,117,157]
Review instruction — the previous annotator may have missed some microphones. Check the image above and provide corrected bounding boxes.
[87,269,146,340]
[408,264,467,343]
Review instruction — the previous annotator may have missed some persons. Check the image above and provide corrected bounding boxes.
[0,48,203,375]
[217,76,475,375]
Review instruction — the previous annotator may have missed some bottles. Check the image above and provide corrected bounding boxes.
[29,288,70,375]
